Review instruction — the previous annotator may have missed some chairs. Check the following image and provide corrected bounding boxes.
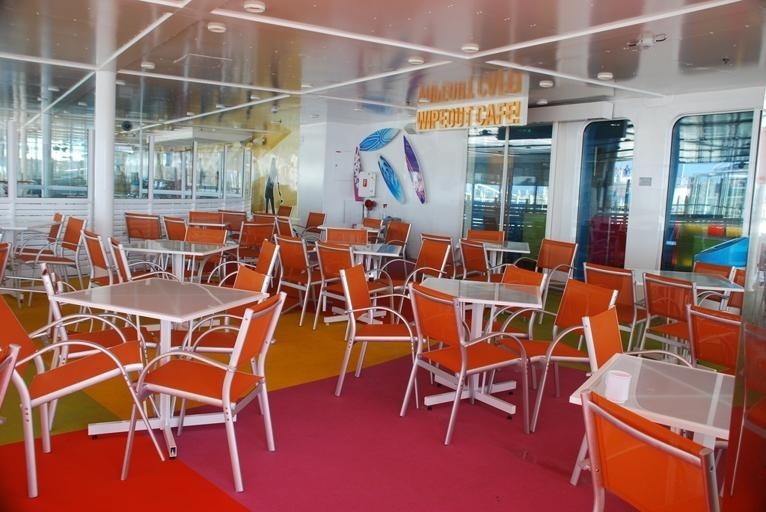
[0,204,454,297]
[580,385,720,512]
[513,239,579,323]
[467,266,551,390]
[312,240,353,328]
[2,299,164,498]
[334,264,426,395]
[457,238,504,283]
[169,266,275,352]
[1,344,21,403]
[487,278,621,432]
[40,265,156,434]
[272,233,322,328]
[580,261,651,357]
[683,304,743,383]
[638,271,699,362]
[465,229,505,244]
[582,305,621,375]
[723,268,747,314]
[122,293,286,490]
[691,262,733,310]
[400,284,530,445]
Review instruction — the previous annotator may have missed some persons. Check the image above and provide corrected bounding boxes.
[264,156,282,215]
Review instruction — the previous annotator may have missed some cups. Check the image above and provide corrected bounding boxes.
[604,370,631,403]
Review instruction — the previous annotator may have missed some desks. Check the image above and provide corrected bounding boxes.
[53,277,269,457]
[485,241,531,273]
[632,270,743,359]
[419,277,542,416]
[571,353,735,486]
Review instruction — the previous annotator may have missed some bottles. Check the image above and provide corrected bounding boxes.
[381,204,389,219]
